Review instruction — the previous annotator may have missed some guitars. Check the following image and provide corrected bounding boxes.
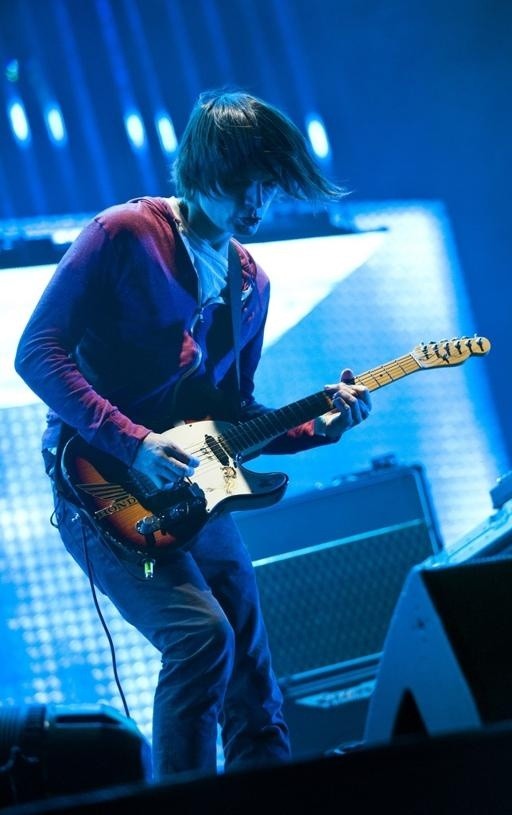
[57,333,491,570]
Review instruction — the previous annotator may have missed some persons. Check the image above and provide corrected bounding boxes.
[13,90,372,780]
